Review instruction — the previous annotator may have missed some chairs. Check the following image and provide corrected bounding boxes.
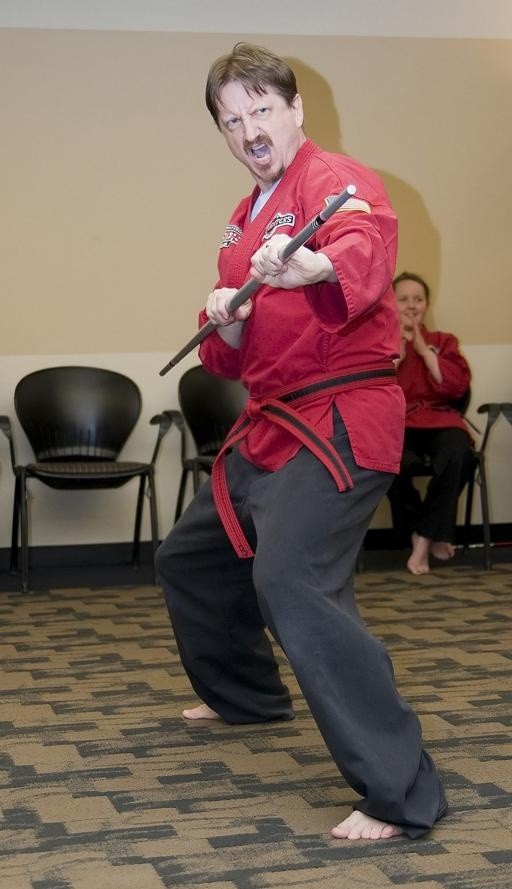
[163,365,249,525]
[354,385,500,574]
[1,363,174,595]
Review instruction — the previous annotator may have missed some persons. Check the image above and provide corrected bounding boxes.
[386,273,477,576]
[150,37,449,844]
